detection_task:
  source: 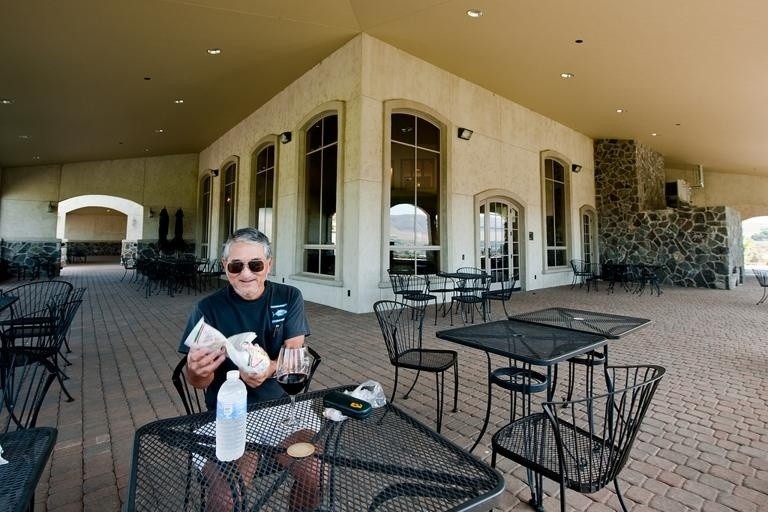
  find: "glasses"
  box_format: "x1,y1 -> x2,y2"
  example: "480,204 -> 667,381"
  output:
227,259 -> 265,274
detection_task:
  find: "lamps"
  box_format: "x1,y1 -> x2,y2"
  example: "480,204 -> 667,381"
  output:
571,163 -> 581,173
210,168 -> 218,177
457,126 -> 472,140
147,206 -> 153,218
278,131 -> 291,144
46,200 -> 53,212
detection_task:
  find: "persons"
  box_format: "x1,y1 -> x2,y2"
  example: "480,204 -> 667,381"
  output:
178,227 -> 329,512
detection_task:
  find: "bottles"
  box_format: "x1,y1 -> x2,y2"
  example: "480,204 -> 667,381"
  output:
215,369 -> 248,462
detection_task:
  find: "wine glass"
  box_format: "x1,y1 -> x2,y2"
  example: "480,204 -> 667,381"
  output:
276,343 -> 311,426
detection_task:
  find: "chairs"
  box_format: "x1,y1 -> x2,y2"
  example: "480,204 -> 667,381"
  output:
120,249 -> 224,300
2,281 -> 87,430
172,343 -> 322,512
491,365 -> 665,510
570,259 -> 664,298
387,267 -> 492,328
482,273 -> 519,320
751,266 -> 767,308
372,300 -> 458,434
